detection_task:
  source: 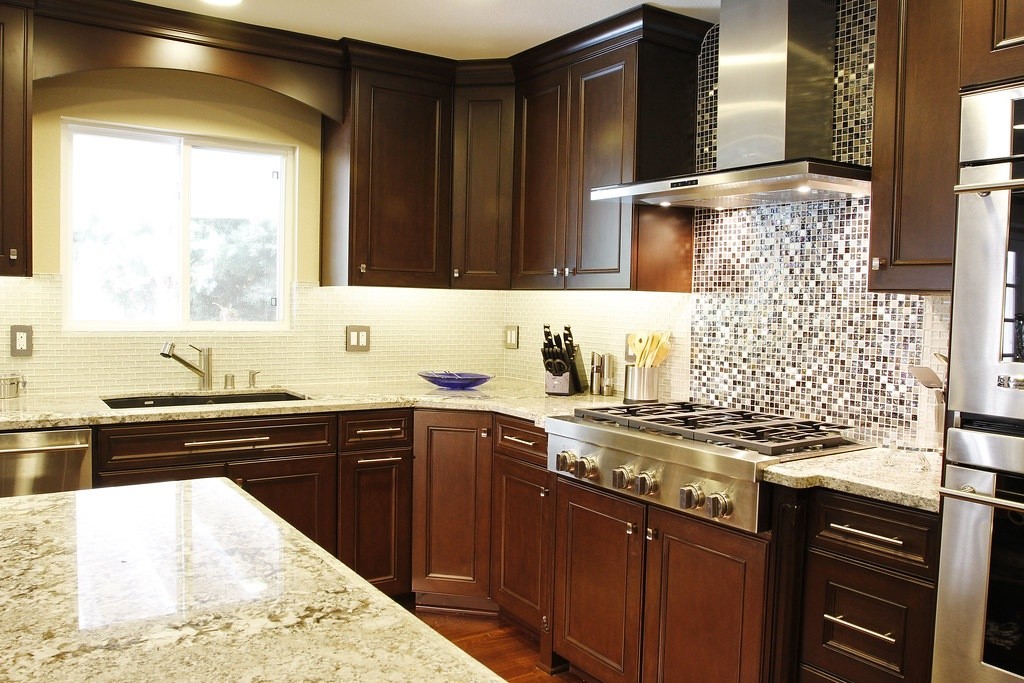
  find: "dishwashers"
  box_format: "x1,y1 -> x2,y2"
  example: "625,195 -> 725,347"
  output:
0,426 -> 93,497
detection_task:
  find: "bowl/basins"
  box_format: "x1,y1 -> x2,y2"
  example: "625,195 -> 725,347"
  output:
417,372 -> 492,389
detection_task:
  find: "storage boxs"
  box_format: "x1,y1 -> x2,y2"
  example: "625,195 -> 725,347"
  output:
543,344 -> 579,395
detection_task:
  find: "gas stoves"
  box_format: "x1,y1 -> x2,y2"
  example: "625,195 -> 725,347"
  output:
544,400 -> 856,534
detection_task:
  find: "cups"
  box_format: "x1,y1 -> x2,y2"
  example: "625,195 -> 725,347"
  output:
623,365 -> 659,404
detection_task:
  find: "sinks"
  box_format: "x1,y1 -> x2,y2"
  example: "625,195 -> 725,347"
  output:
100,386 -> 312,409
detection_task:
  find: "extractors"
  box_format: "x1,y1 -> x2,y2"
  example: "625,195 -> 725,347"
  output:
590,156 -> 871,210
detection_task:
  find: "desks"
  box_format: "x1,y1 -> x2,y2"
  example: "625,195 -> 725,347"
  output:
0,476 -> 510,683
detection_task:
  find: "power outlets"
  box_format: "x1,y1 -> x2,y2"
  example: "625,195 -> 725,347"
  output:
624,333 -> 637,363
504,325 -> 519,349
346,325 -> 370,352
10,325 -> 32,357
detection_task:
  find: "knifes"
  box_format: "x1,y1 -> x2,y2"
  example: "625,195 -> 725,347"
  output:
540,323 -> 575,371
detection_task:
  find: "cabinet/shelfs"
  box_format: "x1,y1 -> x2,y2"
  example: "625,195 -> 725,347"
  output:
491,412 -> 570,674
552,475 -> 775,683
336,409 -> 413,595
866,0 -> 962,296
449,59 -> 514,292
316,37 -> 452,291
957,0 -> 1024,92
794,487 -> 939,683
0,0 -> 33,279
510,2 -> 718,294
92,410 -> 338,558
410,407 -> 499,618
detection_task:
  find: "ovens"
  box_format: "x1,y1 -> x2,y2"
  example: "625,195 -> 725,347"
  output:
930,78 -> 1024,683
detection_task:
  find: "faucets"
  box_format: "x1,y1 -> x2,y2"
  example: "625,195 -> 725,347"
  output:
249,370 -> 260,387
160,340 -> 213,390
